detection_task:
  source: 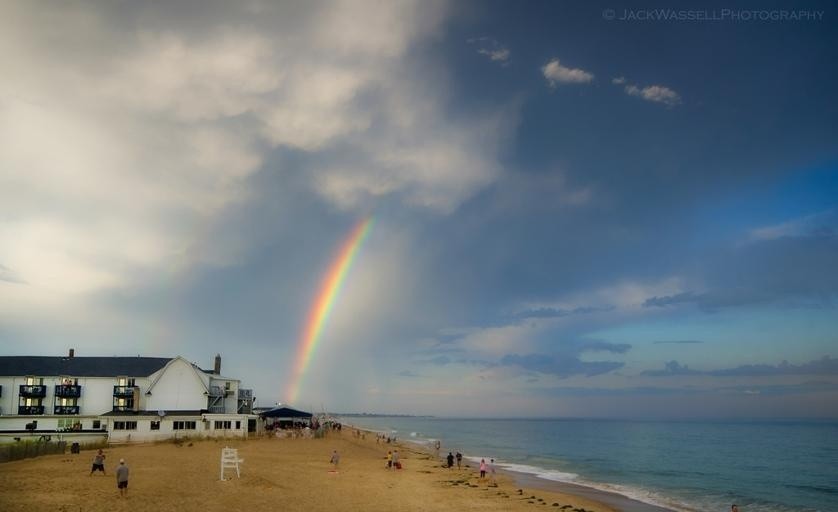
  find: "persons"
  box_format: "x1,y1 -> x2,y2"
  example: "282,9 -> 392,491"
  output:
329,449 -> 340,472
479,458 -> 497,485
88,449 -> 107,480
385,450 -> 402,473
447,451 -> 462,472
263,415 -> 341,440
351,424 -> 397,444
116,458 -> 129,499
